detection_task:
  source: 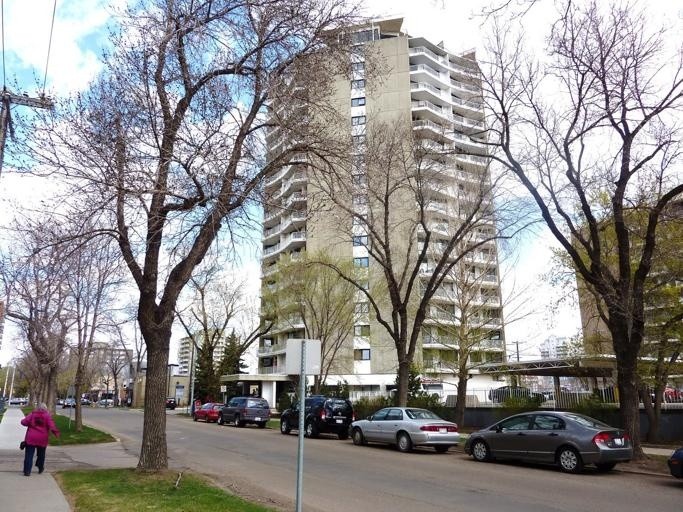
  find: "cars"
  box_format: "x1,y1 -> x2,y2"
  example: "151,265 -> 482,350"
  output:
347,407 -> 463,453
9,394 -> 116,409
668,448 -> 683,479
550,387 -> 570,399
489,385 -> 546,407
193,402 -> 224,423
651,387 -> 683,403
463,410 -> 634,474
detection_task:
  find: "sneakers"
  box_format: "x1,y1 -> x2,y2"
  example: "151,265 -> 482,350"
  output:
40,468 -> 43,473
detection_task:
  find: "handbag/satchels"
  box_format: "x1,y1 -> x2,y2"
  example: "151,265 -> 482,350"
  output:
21,442 -> 25,449
24,473 -> 29,476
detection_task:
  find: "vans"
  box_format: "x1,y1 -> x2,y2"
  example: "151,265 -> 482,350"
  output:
218,396 -> 271,428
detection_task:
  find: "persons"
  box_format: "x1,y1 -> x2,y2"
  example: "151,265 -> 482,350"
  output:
253,388 -> 259,397
19,402 -> 60,476
190,400 -> 196,419
252,389 -> 255,395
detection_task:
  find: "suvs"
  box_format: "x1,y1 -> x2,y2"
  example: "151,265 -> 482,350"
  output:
280,394 -> 354,440
165,397 -> 177,410
